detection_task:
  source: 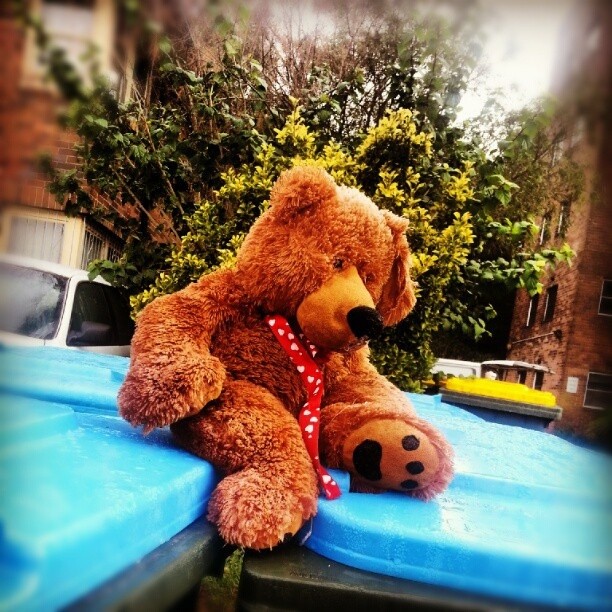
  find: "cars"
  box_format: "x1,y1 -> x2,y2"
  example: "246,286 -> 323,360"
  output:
0,251 -> 134,358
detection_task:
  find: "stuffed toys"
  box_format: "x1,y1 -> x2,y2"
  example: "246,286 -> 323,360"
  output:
116,163 -> 455,551
